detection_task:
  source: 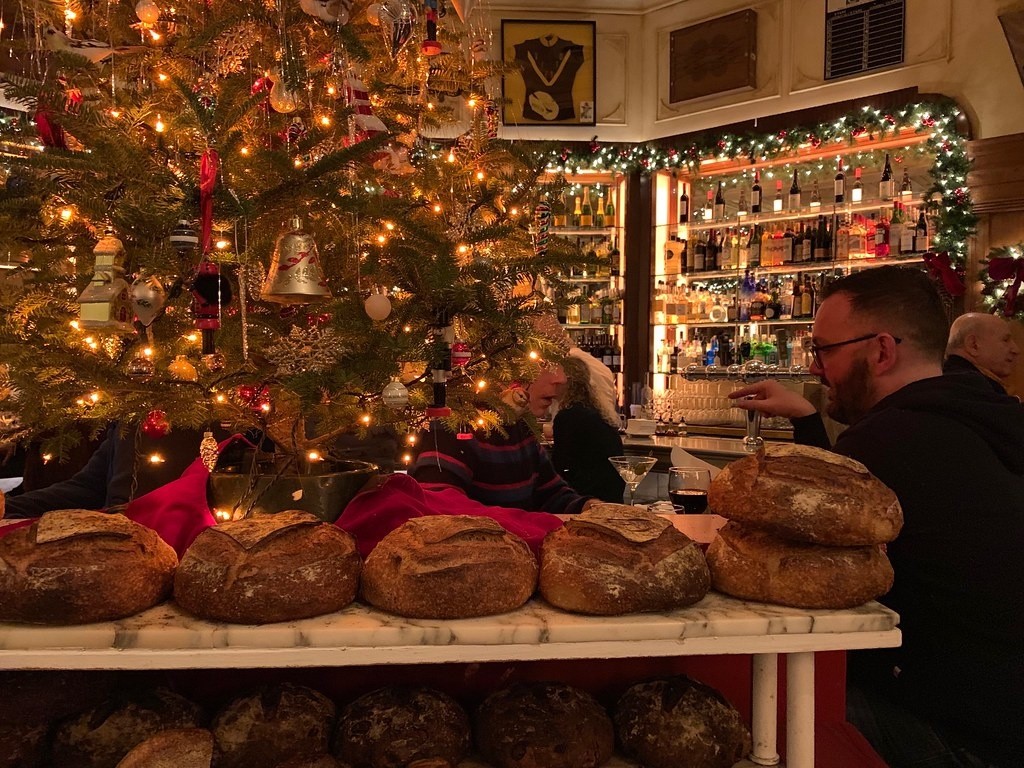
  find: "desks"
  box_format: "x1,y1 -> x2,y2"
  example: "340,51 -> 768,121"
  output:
1,597 -> 905,767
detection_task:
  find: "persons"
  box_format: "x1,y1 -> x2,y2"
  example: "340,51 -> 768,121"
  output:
409,307 -> 625,512
0,416 -> 277,520
728,267 -> 1024,768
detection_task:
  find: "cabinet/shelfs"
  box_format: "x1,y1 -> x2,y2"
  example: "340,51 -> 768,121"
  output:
647,129 -> 961,441
529,170 -> 627,427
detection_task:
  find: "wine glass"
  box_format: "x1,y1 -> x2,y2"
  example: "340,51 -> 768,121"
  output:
646,375 -> 747,428
608,456 -> 658,507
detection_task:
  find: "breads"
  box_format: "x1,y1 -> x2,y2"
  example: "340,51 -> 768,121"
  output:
0,672 -> 755,768
703,442 -> 902,610
0,504 -> 713,626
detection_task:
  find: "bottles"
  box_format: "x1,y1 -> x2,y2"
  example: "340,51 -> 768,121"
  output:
837,202 -> 892,261
704,167 -> 918,220
739,190 -> 748,211
713,181 -> 725,224
657,326 -> 814,375
751,171 -> 762,213
574,333 -> 622,373
788,169 -> 802,213
545,284 -> 621,327
834,159 -> 846,204
613,373 -> 687,437
538,186 -> 615,229
680,183 -> 689,223
651,265 -> 854,324
534,235 -> 620,280
665,216 -> 832,274
879,154 -> 894,201
889,201 -> 944,258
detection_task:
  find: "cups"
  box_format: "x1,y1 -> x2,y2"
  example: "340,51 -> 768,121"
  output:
669,467 -> 711,515
647,504 -> 685,515
630,405 -> 640,419
543,425 -> 554,441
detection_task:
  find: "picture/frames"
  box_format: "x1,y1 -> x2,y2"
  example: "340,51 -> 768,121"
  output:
669,9 -> 759,105
501,17 -> 596,127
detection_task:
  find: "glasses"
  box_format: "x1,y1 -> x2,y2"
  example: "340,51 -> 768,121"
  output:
808,334 -> 903,370
